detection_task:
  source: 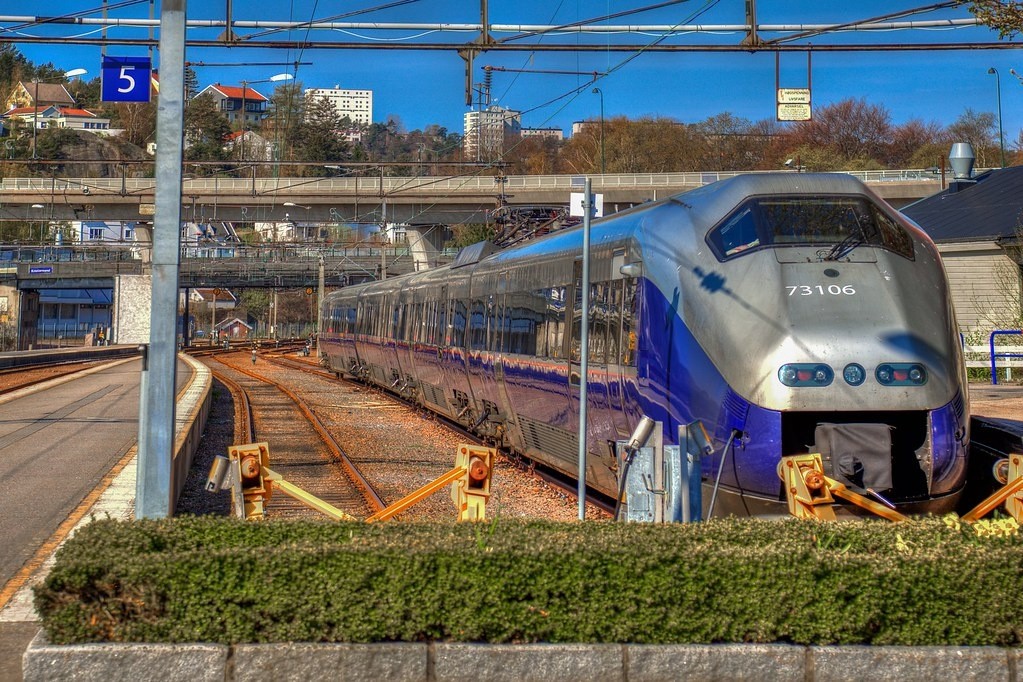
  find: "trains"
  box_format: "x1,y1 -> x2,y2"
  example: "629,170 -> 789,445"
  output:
314,172 -> 972,520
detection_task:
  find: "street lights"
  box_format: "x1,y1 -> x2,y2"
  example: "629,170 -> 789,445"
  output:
592,87 -> 606,174
241,73 -> 295,162
28,68 -> 89,162
987,67 -> 1005,167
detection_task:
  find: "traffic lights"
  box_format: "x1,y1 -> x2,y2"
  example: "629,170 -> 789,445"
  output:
212,287 -> 221,297
306,288 -> 313,295
